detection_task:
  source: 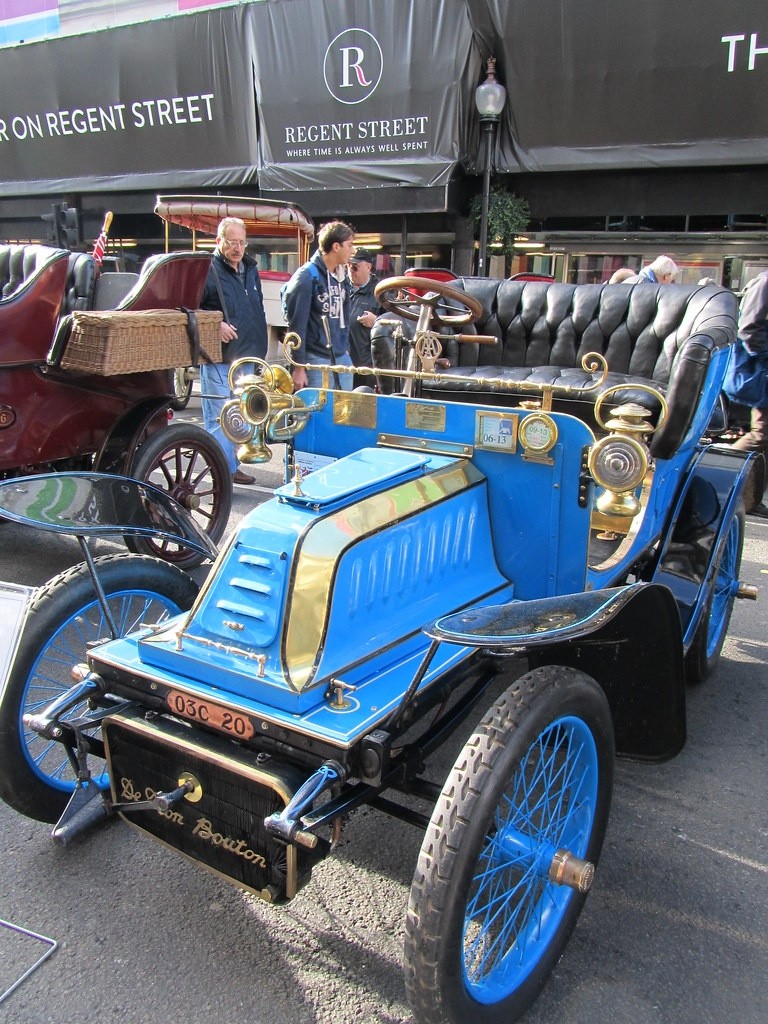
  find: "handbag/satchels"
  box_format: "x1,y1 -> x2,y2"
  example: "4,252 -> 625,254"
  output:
722,340 -> 768,408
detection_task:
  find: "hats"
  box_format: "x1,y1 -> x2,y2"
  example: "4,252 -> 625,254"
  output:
347,247 -> 372,264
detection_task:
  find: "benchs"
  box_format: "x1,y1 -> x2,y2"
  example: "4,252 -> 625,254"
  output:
0,244 -> 71,368
114,250 -> 213,310
371,275 -> 739,459
59,251 -> 98,319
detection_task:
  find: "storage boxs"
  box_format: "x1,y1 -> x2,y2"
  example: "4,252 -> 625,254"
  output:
62,309 -> 223,375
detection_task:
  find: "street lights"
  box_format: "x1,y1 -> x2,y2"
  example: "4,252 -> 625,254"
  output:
474,55 -> 507,279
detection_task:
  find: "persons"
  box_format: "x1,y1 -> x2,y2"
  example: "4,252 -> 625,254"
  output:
345,249 -> 396,390
698,270 -> 768,518
602,254 -> 679,283
283,218 -> 356,390
199,215 -> 272,487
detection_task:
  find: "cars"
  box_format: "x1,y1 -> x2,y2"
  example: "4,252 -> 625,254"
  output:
0,276 -> 768,1024
0,244 -> 227,572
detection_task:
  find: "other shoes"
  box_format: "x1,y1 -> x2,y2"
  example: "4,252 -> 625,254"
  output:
233,470 -> 256,484
745,503 -> 768,518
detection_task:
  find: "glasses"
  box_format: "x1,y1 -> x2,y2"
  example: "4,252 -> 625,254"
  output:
346,263 -> 368,271
225,239 -> 248,247
